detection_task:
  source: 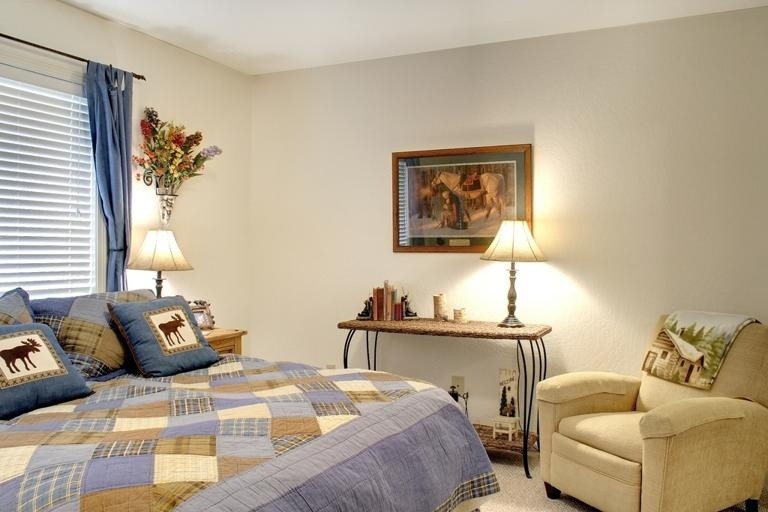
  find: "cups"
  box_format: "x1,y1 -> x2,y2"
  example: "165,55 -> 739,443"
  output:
433,294 -> 445,320
452,307 -> 466,321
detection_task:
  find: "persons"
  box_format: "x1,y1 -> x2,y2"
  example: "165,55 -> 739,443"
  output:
441,190 -> 462,230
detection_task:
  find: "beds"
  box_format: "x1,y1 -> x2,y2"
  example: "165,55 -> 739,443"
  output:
1,277 -> 504,512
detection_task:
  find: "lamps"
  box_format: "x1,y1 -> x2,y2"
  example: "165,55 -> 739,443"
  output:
478,217 -> 546,330
128,228 -> 195,298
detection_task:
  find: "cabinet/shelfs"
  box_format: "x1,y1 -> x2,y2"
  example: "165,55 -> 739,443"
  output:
201,326 -> 250,355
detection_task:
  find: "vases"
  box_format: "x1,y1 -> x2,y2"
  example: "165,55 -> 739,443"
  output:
159,193 -> 175,226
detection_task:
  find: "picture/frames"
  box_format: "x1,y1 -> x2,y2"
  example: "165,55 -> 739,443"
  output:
386,141 -> 535,258
191,306 -> 216,331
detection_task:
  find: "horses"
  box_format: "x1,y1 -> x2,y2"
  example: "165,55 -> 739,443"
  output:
431,170 -> 506,221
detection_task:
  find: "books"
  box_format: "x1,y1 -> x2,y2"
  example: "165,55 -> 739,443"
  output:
372,279 -> 407,321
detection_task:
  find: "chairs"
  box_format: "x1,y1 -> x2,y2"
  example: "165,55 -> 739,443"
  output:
532,304 -> 767,511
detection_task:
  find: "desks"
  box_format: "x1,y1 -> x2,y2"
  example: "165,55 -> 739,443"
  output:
336,314 -> 550,480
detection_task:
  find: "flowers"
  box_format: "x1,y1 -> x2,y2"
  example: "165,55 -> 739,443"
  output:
128,102 -> 224,195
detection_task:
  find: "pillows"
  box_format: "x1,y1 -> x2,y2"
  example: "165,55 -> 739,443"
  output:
31,288 -> 155,380
101,293 -> 223,376
2,320 -> 94,418
0,286 -> 34,327
